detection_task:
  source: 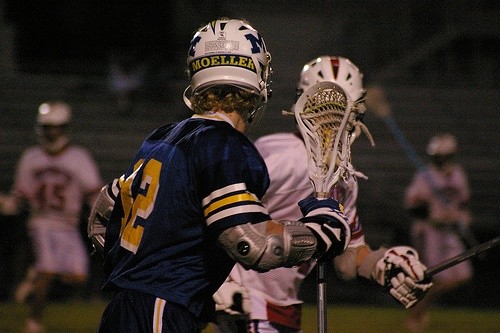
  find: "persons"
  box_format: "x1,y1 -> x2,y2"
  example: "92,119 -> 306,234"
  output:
404,133 -> 473,331
87,16 -> 350,332
212,54 -> 432,332
1,98 -> 103,332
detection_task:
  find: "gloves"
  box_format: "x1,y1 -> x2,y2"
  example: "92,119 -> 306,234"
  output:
373,245 -> 433,310
298,197 -> 351,262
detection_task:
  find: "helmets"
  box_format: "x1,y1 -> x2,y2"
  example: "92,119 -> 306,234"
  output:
34,102 -> 71,153
185,18 -> 271,110
428,135 -> 457,172
296,55 -> 367,144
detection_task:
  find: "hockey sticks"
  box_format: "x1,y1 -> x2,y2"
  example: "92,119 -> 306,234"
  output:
423,237 -> 500,278
293,80 -> 356,333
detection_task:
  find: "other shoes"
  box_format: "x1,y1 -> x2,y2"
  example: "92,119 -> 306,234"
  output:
27,317 -> 41,333
16,270 -> 39,306
